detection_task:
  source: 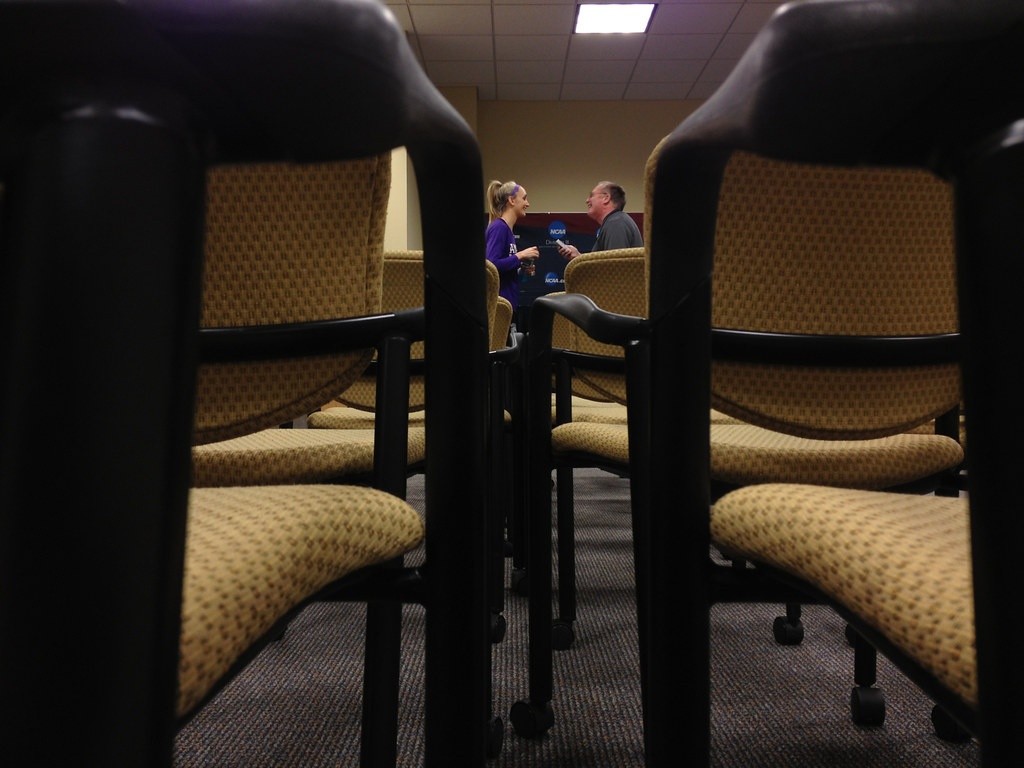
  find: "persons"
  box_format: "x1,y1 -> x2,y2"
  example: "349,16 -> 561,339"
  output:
485,179 -> 540,348
558,180 -> 644,258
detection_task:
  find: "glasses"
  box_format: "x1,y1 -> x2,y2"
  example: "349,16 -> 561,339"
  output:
588,192 -> 608,198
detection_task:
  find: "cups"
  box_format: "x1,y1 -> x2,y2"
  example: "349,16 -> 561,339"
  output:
521,258 -> 534,269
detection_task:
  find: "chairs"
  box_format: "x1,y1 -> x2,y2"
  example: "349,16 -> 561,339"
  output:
1,0 -> 1024,768
